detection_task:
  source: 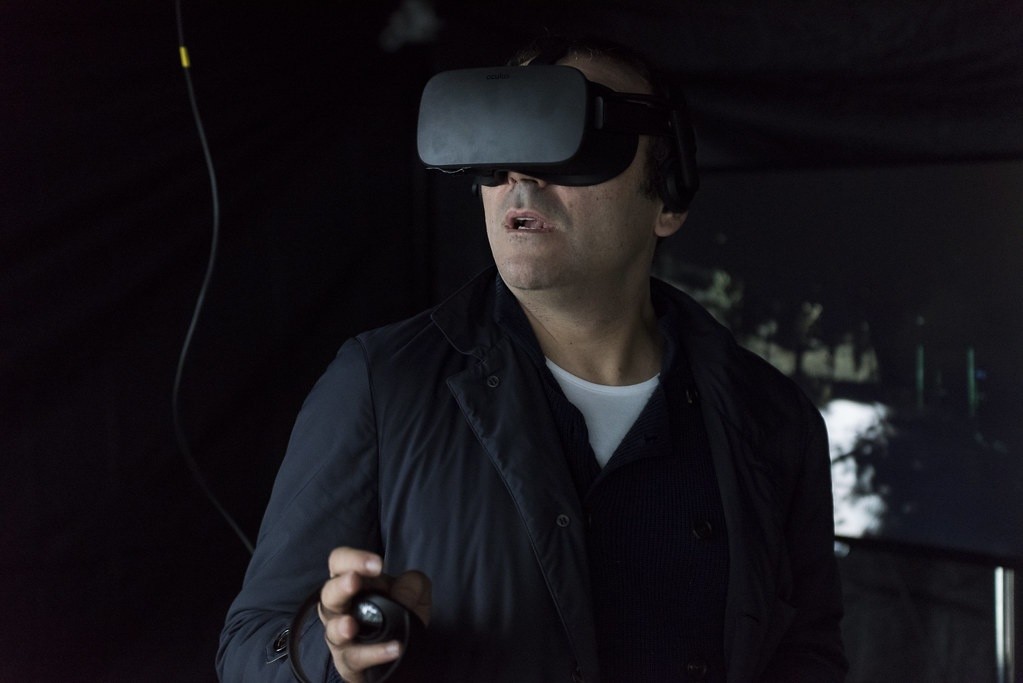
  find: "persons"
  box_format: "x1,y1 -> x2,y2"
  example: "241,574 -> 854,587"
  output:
213,24 -> 849,682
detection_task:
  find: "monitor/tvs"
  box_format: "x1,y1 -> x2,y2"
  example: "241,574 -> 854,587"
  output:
651,151 -> 1022,572
